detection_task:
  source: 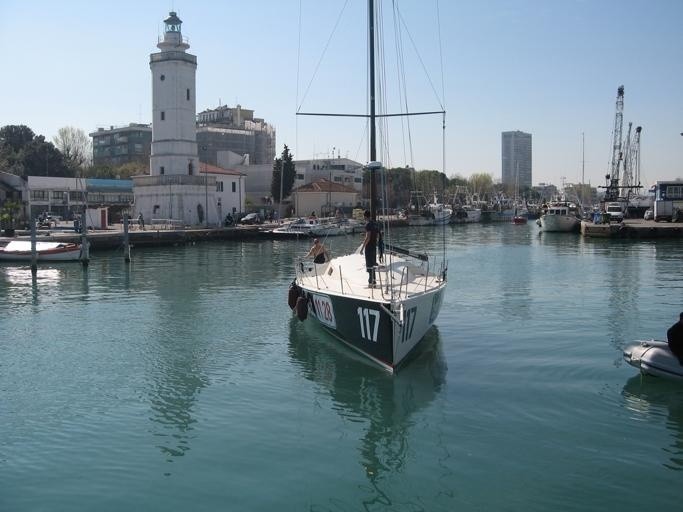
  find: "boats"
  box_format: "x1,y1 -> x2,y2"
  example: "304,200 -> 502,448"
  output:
0,240 -> 89,261
622,339 -> 682,380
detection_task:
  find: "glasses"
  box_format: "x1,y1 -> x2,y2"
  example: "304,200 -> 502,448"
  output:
314,241 -> 319,244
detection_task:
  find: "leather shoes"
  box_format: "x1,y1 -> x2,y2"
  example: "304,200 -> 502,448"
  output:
368,284 -> 376,287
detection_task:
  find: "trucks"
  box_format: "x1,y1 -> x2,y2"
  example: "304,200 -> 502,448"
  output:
652,200 -> 672,222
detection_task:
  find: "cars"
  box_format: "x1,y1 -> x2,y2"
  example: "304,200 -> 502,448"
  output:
603,205 -> 624,223
240,213 -> 264,225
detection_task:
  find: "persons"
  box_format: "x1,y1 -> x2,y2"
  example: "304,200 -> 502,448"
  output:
224,212 -> 233,226
298,237 -> 329,276
359,209 -> 382,291
273,209 -> 278,223
310,211 -> 317,224
335,207 -> 344,227
666,312 -> 683,367
136,212 -> 145,230
